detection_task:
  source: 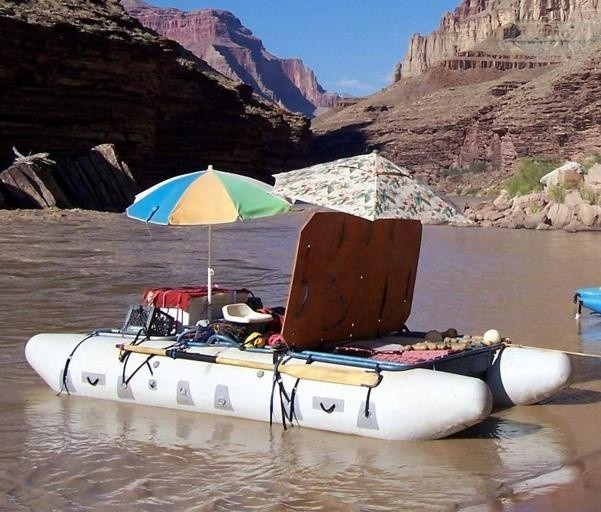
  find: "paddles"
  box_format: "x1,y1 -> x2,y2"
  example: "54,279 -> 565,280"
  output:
116,342 -> 383,388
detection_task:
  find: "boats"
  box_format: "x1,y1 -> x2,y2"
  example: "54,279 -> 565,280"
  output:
25,304 -> 574,442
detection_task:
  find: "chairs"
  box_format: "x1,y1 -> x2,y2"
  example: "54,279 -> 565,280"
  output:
222,302 -> 274,324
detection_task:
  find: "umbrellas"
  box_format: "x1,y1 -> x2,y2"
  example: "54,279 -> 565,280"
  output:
267,148 -> 480,230
125,165 -> 296,322
